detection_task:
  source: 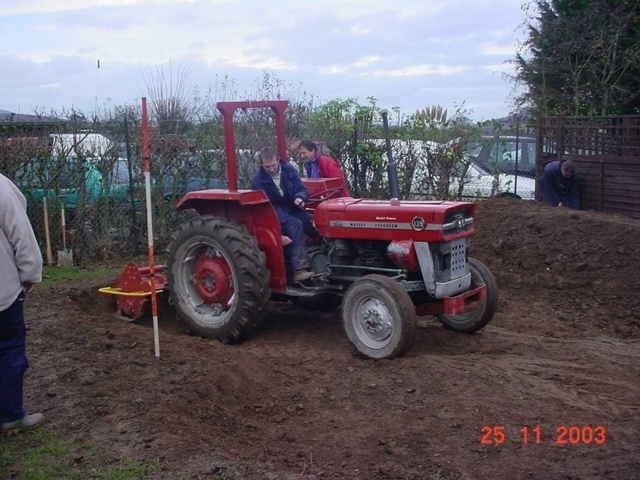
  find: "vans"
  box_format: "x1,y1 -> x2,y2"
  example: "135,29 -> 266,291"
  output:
161,149 -> 261,200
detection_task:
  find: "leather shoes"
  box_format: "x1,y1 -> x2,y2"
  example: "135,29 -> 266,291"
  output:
0,412 -> 45,433
293,270 -> 315,282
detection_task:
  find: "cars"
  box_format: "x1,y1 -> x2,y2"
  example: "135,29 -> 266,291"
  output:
14,155 -> 157,226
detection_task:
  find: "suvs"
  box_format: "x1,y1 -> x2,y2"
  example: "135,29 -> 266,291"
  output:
442,135 -> 571,176
343,138 -> 534,201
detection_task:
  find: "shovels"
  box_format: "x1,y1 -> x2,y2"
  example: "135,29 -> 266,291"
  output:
56,202 -> 74,267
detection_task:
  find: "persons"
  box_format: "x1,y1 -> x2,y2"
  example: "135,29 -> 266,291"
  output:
0,171 -> 48,437
536,157 -> 580,210
250,146 -> 322,284
297,139 -> 351,198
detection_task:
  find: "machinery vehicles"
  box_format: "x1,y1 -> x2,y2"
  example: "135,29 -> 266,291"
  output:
166,101 -> 498,359
